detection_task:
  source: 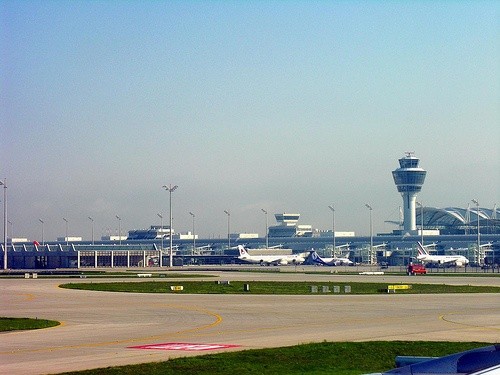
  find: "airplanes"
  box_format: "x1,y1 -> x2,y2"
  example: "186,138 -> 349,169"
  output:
416,241 -> 470,267
307,248 -> 354,266
237,245 -> 306,266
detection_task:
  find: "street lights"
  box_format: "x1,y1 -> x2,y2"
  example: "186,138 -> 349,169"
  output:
365,203 -> 374,265
328,205 -> 335,258
416,201 -> 424,247
157,213 -> 164,268
224,209 -> 231,248
261,208 -> 268,248
0,176 -> 8,269
162,184 -> 179,266
63,217 -> 69,246
472,199 -> 481,265
189,211 -> 196,255
116,215 -> 122,246
88,215 -> 95,245
38,218 -> 44,245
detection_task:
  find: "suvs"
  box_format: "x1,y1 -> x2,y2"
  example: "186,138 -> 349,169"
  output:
406,264 -> 427,275
